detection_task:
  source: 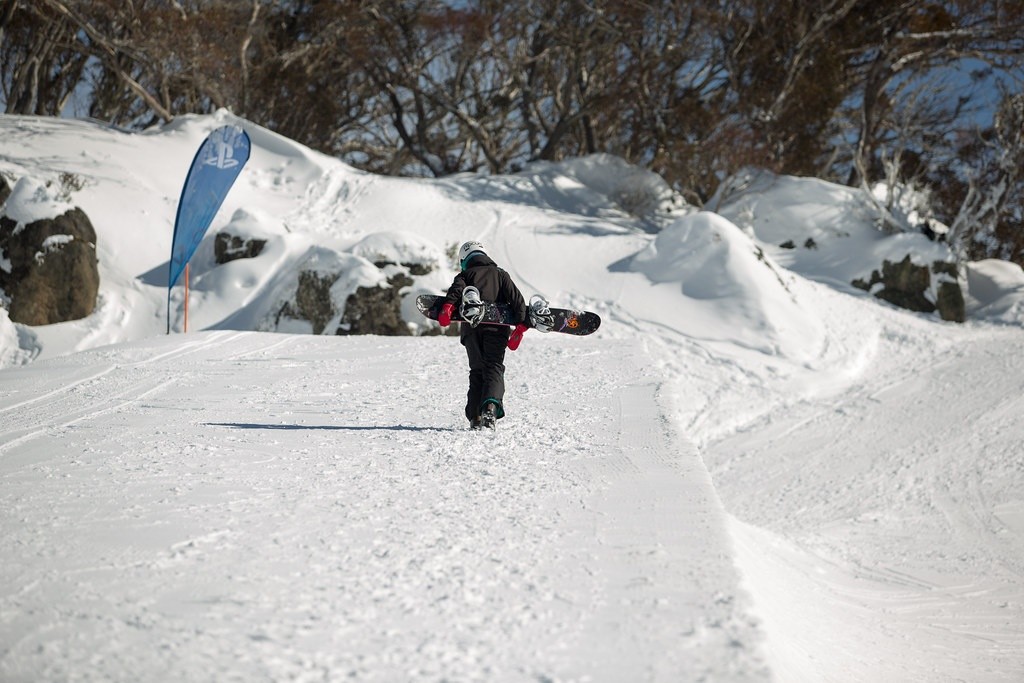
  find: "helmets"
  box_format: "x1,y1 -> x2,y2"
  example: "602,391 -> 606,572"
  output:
456,241 -> 486,266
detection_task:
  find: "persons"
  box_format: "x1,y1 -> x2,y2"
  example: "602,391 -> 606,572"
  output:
438,241 -> 530,429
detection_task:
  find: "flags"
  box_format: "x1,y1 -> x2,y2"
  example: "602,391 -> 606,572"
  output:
168,124 -> 252,288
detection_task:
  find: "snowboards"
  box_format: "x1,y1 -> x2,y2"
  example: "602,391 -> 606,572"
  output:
415,286 -> 601,337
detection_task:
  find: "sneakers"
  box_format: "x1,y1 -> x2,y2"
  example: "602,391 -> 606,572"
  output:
480,404 -> 495,431
470,414 -> 480,430
